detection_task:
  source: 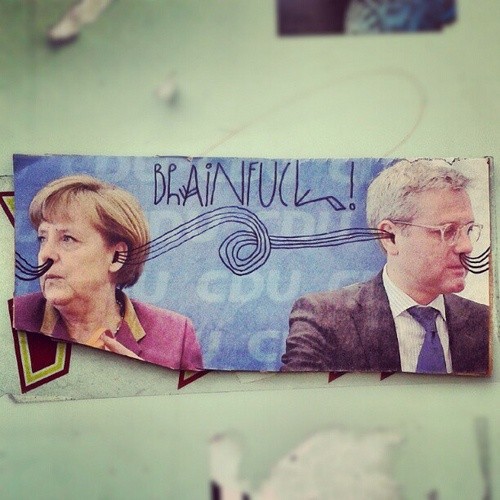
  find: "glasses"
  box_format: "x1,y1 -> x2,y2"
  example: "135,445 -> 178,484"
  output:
388,215 -> 485,248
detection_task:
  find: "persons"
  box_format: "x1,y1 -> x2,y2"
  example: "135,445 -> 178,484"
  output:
13,174 -> 203,371
278,159 -> 489,375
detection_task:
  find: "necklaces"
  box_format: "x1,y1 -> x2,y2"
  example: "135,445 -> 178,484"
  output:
113,299 -> 125,338
